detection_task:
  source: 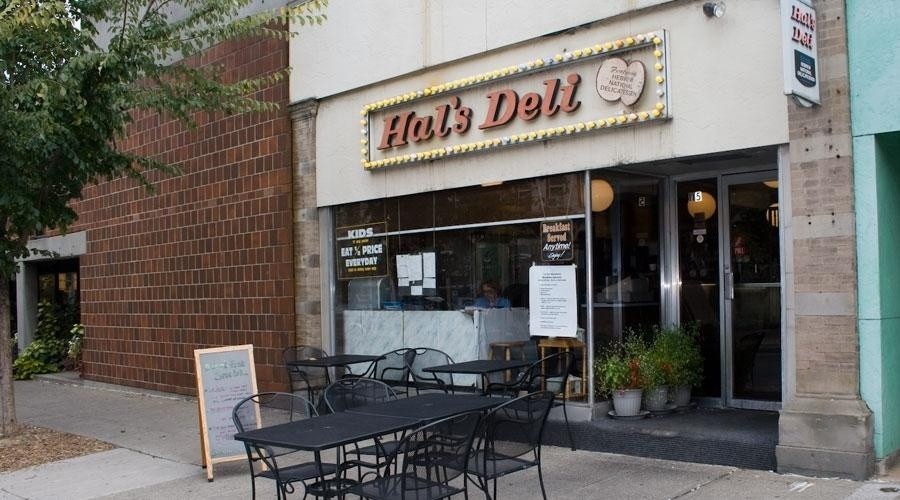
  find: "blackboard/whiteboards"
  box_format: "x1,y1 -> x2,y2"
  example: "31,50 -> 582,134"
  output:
194,343 -> 266,463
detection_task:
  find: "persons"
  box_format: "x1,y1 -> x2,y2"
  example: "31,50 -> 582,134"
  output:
472,280 -> 511,309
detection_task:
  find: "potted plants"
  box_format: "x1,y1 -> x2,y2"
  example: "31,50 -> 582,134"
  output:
593,318 -> 707,422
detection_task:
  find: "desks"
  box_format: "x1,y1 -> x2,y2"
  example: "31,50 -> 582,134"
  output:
537,340 -> 586,402
